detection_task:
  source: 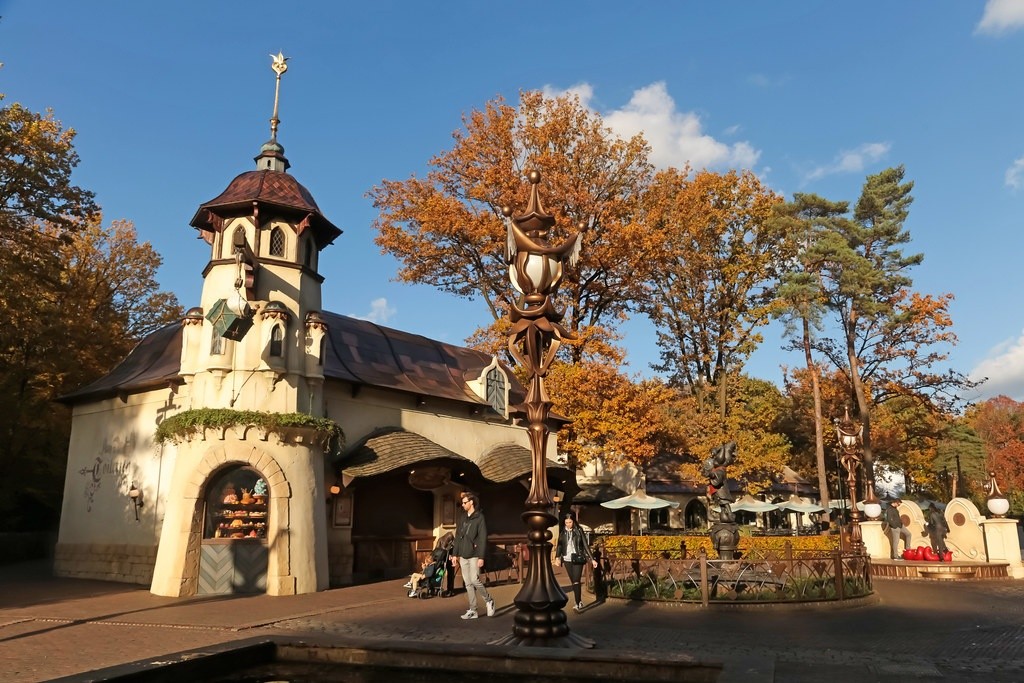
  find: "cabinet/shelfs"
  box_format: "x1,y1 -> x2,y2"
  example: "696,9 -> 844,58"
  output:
217,504 -> 267,529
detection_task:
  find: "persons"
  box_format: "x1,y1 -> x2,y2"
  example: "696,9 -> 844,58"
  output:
859,512 -> 867,521
435,533 -> 454,596
697,441 -> 737,522
555,513 -> 597,613
776,520 -> 784,529
887,499 -> 911,561
452,495 -> 496,619
925,504 -> 950,560
1009,512 -> 1024,532
403,557 -> 434,597
835,513 -> 843,527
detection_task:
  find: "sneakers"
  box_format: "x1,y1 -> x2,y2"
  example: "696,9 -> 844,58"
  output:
486,599 -> 495,616
460,608 -> 478,619
409,590 -> 417,597
403,582 -> 412,588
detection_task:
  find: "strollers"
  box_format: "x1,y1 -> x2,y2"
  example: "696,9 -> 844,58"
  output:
407,548 -> 452,600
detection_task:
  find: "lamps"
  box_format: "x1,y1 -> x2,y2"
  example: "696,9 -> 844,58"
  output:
864,479 -> 882,521
130,481 -> 144,521
329,470 -> 341,495
987,470 -> 1010,518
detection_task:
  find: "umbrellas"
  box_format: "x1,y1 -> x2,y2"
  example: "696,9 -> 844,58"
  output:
819,500 -> 947,510
776,497 -> 826,534
712,494 -> 780,525
601,490 -> 680,536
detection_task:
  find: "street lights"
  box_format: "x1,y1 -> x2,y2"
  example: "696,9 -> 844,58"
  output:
485,168 -> 602,650
834,406 -> 869,555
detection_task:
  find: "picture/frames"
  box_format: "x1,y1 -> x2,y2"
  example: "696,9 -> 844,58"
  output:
332,493 -> 353,528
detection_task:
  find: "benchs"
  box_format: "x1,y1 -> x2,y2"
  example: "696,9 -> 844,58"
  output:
480,540 -> 516,585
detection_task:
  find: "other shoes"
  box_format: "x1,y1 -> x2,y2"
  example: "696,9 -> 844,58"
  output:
445,590 -> 455,596
573,605 -> 580,614
579,601 -> 584,609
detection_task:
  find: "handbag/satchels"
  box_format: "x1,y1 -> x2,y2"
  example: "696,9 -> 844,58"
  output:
571,553 -> 587,565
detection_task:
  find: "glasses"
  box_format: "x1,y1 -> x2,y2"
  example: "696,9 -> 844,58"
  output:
462,501 -> 470,505
